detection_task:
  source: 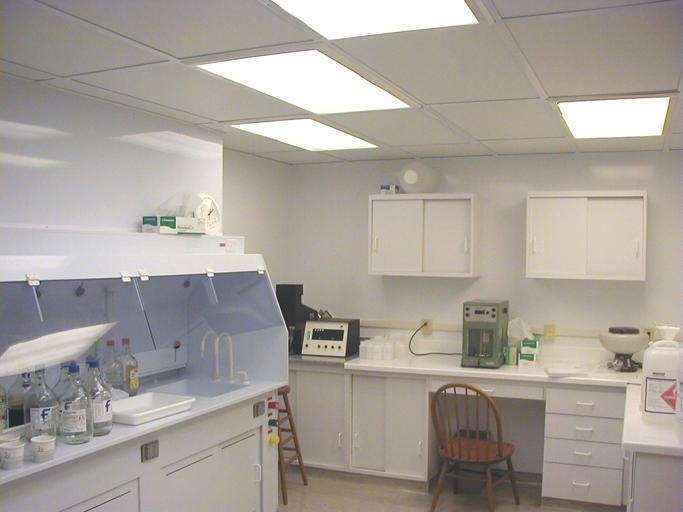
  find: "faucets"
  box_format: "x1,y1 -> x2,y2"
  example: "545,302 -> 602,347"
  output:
200,330 -> 220,376
214,332 -> 234,379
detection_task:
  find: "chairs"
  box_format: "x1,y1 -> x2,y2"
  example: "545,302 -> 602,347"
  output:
430,382 -> 520,512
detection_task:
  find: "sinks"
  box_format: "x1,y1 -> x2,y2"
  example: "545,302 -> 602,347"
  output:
144,378 -> 247,399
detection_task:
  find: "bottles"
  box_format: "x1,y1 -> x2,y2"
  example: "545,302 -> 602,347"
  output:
0,336 -> 140,445
357,334 -> 406,362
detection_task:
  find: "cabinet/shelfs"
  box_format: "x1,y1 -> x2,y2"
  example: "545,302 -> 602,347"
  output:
542,385 -> 626,508
368,195 -> 475,279
1,405 -> 268,511
527,192 -> 646,280
281,369 -> 443,481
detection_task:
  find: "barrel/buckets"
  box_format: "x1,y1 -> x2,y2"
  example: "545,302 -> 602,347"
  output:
640,340 -> 682,421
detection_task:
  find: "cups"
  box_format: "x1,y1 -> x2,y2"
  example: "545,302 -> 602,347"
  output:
0,432 -> 24,470
29,435 -> 56,464
